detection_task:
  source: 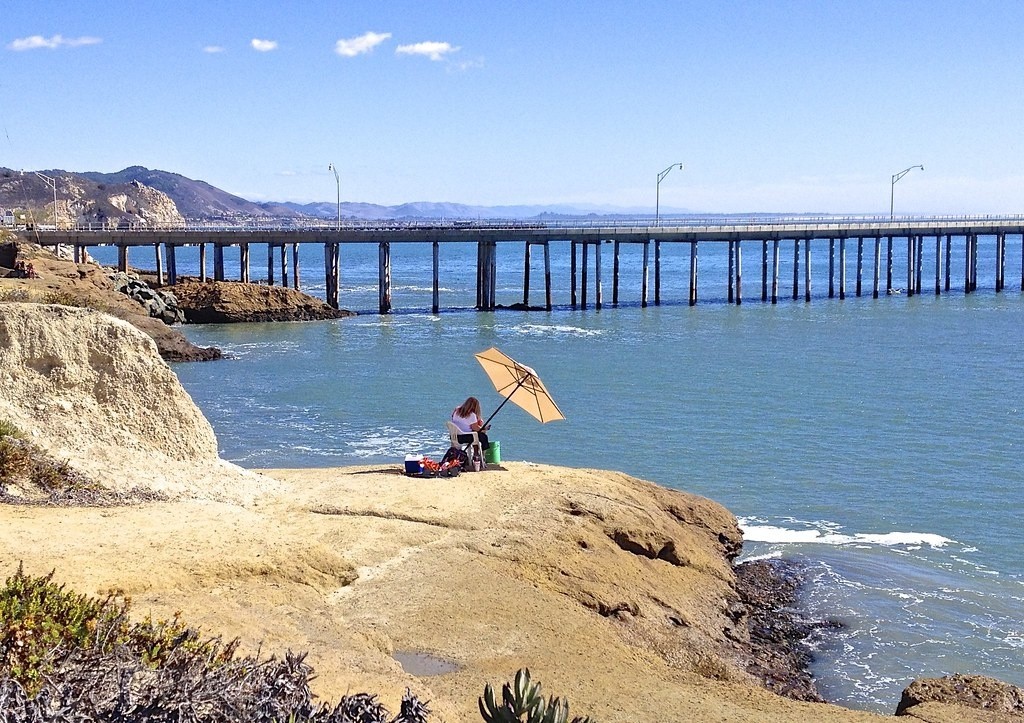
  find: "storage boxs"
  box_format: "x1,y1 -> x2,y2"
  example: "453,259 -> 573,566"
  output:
405,453 -> 423,474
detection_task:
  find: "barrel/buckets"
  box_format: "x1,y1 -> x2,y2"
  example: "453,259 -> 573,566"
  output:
485,441 -> 501,464
405,454 -> 424,475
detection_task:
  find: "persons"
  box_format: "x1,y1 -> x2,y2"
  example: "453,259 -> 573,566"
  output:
450,397 -> 489,468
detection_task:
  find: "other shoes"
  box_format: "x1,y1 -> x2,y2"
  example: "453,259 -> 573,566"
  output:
482,462 -> 489,468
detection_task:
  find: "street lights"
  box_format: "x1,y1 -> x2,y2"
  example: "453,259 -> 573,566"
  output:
890,165 -> 924,223
655,162 -> 683,227
328,163 -> 341,231
19,167 -> 58,231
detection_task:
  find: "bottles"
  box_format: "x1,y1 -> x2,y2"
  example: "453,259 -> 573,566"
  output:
474,457 -> 481,472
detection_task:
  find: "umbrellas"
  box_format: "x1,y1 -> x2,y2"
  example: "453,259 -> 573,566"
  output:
464,347 -> 566,452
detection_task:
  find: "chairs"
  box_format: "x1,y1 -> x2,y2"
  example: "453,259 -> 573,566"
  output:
446,421 -> 485,469
